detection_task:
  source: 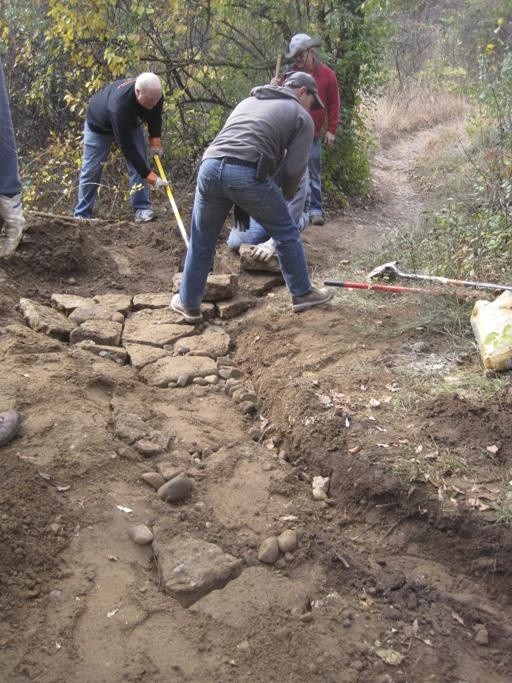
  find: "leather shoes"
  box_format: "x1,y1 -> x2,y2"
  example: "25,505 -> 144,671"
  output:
291,286 -> 334,311
170,292 -> 204,323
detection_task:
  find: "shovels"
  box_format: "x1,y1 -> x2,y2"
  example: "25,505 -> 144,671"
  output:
369,260 -> 512,291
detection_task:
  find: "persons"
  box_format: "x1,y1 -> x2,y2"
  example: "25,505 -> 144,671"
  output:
270,33 -> 340,225
169,71 -> 334,324
227,148 -> 311,262
74,71 -> 172,224
0,70 -> 27,262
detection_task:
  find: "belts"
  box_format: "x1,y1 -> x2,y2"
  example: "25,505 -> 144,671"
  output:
219,156 -> 258,169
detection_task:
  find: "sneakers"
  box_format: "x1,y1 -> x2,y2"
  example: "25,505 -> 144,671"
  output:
311,215 -> 325,225
134,208 -> 155,222
0,410 -> 20,447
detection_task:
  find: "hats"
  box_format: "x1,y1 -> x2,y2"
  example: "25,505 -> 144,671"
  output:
284,72 -> 325,111
285,33 -> 321,59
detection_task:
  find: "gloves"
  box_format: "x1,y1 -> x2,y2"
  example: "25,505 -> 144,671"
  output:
234,204 -> 249,232
0,194 -> 25,257
149,137 -> 163,162
145,172 -> 174,197
250,237 -> 277,263
